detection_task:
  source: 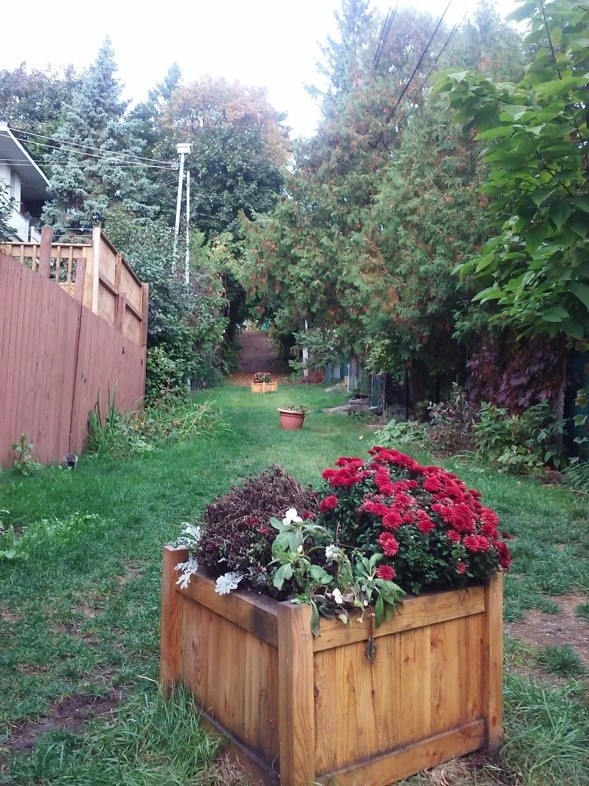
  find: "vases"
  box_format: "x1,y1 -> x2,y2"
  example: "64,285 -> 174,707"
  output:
349,398 -> 367,405
160,544 -> 504,786
251,381 -> 278,394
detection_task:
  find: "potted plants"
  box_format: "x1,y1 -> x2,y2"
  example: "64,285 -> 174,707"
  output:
276,404 -> 308,430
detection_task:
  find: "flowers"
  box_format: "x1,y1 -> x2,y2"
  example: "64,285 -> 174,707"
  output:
162,443 -> 516,637
253,371 -> 272,384
348,393 -> 369,401
